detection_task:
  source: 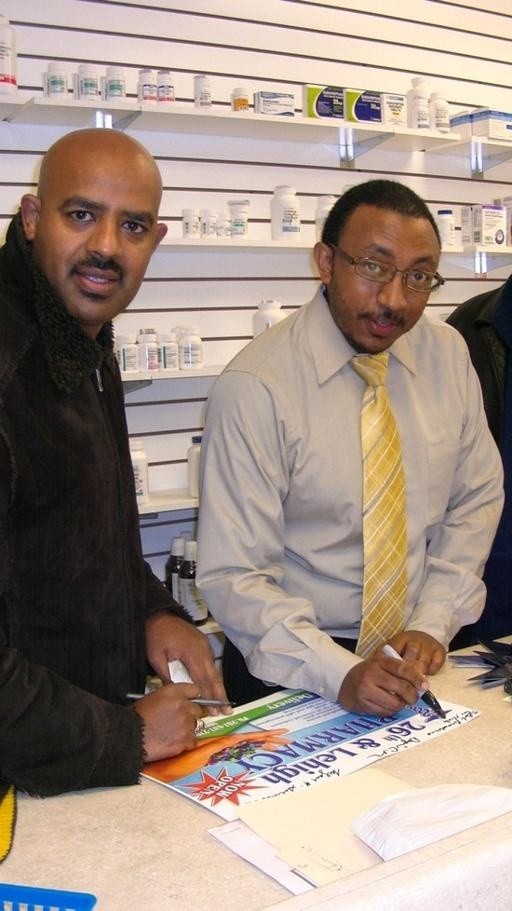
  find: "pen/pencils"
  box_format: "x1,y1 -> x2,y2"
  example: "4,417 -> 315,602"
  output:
382,644 -> 446,719
125,693 -> 236,705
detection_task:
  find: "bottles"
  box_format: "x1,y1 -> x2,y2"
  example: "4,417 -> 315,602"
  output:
185,436 -> 204,503
183,185 -> 338,239
115,327 -> 204,373
407,76 -> 450,131
0,12 -> 250,113
435,208 -> 460,248
167,538 -> 208,625
129,440 -> 147,506
253,299 -> 282,334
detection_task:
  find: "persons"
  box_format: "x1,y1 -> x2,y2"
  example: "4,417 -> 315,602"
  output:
195,181 -> 505,717
0,128 -> 234,797
444,273 -> 512,652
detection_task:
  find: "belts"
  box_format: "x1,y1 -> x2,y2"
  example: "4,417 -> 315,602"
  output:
330,636 -> 357,652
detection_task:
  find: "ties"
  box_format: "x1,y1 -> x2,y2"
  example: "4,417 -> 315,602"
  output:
351,351 -> 408,660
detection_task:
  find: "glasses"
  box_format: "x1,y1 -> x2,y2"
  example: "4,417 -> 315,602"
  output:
330,241 -> 446,293
